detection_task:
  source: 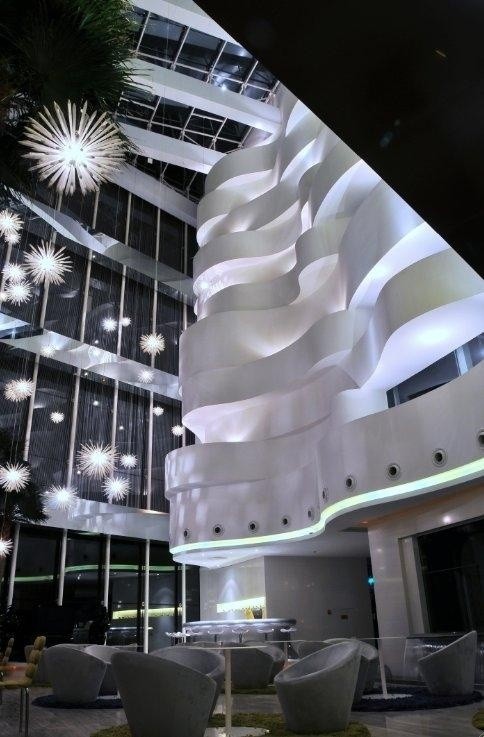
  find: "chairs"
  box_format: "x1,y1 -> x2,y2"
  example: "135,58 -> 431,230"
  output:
296,638 -> 335,657
275,637 -> 363,733
415,626 -> 481,698
325,636 -> 380,695
25,635 -> 287,737
